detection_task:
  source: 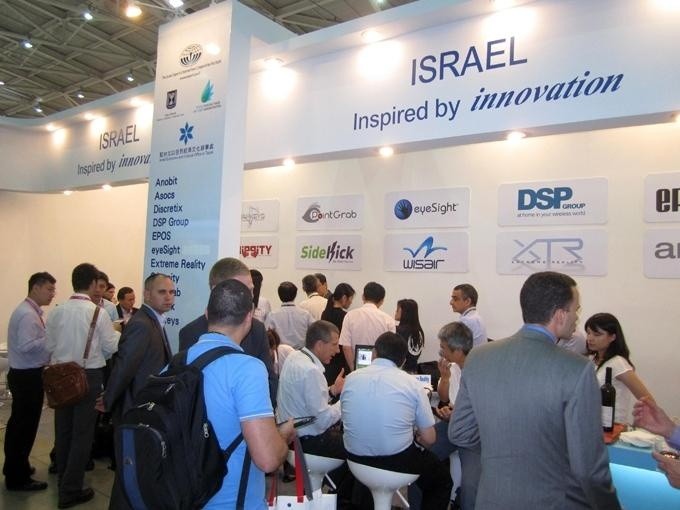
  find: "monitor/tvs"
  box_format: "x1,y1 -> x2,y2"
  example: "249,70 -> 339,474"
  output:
351,343 -> 375,371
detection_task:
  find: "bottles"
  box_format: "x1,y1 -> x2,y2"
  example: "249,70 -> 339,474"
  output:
598,368 -> 615,432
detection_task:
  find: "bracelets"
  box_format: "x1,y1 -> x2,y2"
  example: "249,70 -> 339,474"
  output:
328,386 -> 336,399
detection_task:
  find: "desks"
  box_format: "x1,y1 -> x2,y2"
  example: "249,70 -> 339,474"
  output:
602,423 -> 680,510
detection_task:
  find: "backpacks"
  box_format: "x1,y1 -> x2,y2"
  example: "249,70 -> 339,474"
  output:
109,367 -> 230,510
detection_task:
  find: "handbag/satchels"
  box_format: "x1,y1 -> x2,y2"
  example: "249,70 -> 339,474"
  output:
265,487 -> 338,510
43,360 -> 90,409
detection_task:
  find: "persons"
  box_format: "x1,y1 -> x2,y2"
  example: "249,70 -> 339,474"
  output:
448,271 -> 623,509
276,320 -> 347,460
91,271 -> 119,320
44,263 -> 122,507
408,322 -> 472,509
178,257 -> 277,410
102,283 -> 116,300
312,272 -> 332,298
158,279 -> 298,510
555,329 -> 587,354
265,328 -> 295,376
94,273 -> 175,510
450,284 -> 488,347
116,287 -> 138,320
585,313 -> 658,426
395,299 -> 424,374
264,281 -> 318,348
339,282 -> 396,371
3,271 -> 57,490
632,397 -> 680,489
340,332 -> 453,510
299,274 -> 328,319
321,283 -> 356,386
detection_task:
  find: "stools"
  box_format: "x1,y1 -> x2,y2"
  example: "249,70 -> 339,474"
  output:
347,459 -> 420,510
0,351 -> 13,399
0,356 -> 9,406
284,449 -> 345,496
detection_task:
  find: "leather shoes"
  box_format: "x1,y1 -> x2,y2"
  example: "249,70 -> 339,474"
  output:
2,466 -> 37,477
4,478 -> 49,492
56,488 -> 94,510
